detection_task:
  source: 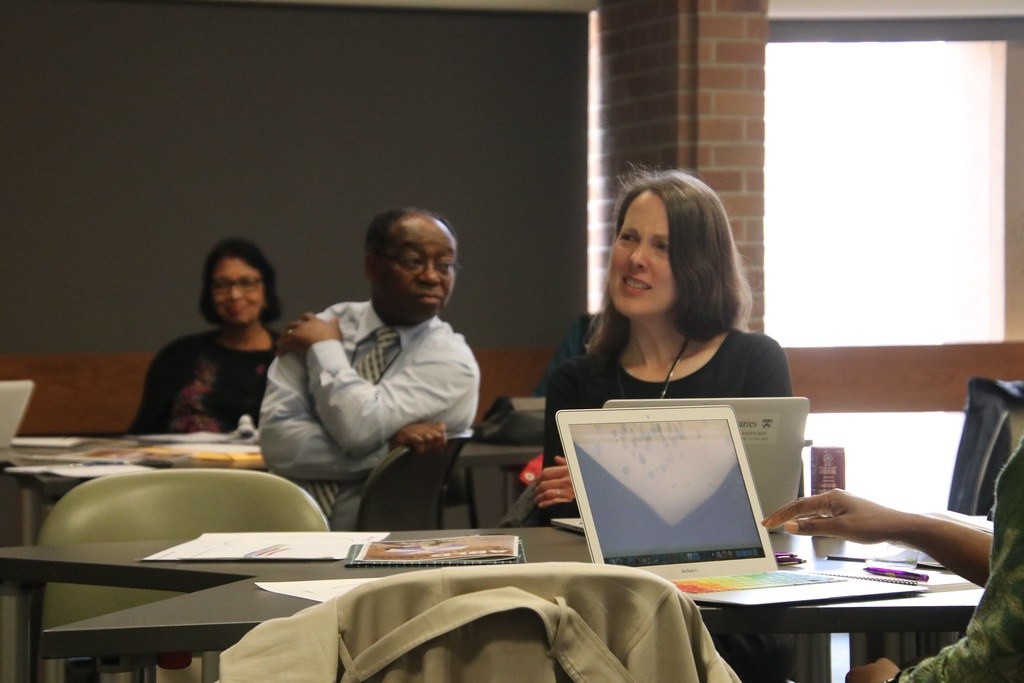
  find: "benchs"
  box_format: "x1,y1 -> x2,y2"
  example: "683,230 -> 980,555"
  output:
0,349 -> 562,435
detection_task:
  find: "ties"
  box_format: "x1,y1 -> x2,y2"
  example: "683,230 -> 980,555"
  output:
301,325 -> 399,518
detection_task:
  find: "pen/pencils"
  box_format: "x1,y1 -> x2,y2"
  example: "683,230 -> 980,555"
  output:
863,567 -> 929,583
776,552 -> 797,558
777,558 -> 807,565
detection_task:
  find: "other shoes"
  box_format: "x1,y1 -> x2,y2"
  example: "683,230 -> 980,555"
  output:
157,651 -> 193,670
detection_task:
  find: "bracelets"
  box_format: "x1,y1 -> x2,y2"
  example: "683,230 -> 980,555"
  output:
888,671 -> 902,683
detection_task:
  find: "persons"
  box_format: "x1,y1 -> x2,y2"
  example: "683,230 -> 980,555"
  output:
258,207 -> 481,531
126,237 -> 282,436
761,438 -> 1024,683
500,170 -> 793,529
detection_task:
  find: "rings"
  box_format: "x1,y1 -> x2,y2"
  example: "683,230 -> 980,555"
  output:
287,329 -> 292,335
557,489 -> 560,497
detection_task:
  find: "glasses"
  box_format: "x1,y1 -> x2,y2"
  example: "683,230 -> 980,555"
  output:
371,249 -> 463,280
213,277 -> 261,295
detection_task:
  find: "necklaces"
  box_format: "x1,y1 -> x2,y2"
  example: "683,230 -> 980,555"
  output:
657,337 -> 689,399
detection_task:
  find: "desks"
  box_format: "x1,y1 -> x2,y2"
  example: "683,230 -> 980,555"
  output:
0,432 -> 544,546
0,524 -> 990,683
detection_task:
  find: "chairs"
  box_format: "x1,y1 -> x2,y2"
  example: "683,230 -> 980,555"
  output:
356,427 -> 475,531
31,467 -> 328,683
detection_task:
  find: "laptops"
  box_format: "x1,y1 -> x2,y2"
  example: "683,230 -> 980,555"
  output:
551,397 -> 929,608
0,379 -> 35,454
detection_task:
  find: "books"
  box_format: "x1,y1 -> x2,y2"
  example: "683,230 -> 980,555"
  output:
344,535 -> 527,569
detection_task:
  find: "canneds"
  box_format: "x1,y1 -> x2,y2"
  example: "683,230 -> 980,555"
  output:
810,446 -> 846,495
